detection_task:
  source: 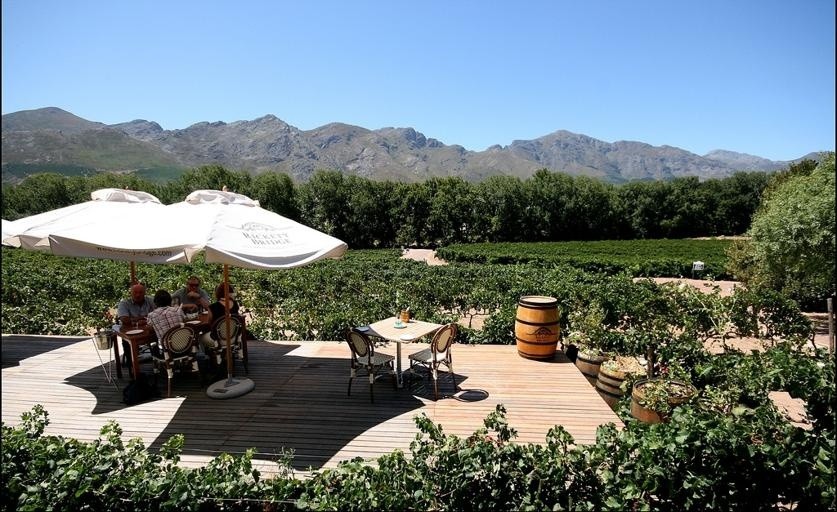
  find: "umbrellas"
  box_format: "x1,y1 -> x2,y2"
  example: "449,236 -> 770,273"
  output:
0,185 -> 167,286
49,186 -> 349,378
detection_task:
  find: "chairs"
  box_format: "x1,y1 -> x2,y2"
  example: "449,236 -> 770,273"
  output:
345,328 -> 397,405
152,325 -> 202,398
122,340 -> 157,368
408,323 -> 460,401
205,314 -> 249,379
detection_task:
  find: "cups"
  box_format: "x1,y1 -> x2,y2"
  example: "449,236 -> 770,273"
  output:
400,309 -> 409,323
132,322 -> 139,330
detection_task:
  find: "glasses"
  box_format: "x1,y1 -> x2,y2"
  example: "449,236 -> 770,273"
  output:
186,281 -> 200,287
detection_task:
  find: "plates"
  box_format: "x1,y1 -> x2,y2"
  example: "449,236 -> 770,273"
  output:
355,327 -> 369,332
394,322 -> 407,329
126,330 -> 144,336
186,320 -> 202,325
400,334 -> 415,341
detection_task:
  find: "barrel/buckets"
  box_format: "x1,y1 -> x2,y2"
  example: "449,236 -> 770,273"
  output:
94,332 -> 113,350
575,353 -> 694,425
515,295 -> 560,361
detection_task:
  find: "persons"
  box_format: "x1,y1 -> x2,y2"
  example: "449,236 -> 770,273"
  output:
116,282 -> 162,371
200,282 -> 239,371
145,290 -> 188,376
169,274 -> 211,310
